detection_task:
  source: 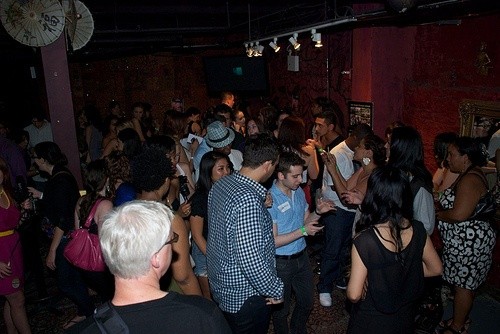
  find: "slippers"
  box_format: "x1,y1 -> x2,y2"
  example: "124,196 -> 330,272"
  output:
63,317 -> 87,330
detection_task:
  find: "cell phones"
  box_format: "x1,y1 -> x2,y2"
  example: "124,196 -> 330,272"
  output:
79,190 -> 87,197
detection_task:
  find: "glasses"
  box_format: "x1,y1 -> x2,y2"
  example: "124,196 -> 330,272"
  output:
165,232 -> 179,244
31,155 -> 41,160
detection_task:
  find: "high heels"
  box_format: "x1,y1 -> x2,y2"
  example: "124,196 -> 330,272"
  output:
435,326 -> 465,334
440,317 -> 470,331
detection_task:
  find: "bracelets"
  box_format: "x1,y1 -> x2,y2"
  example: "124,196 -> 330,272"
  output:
315,209 -> 322,216
190,157 -> 194,159
301,227 -> 307,236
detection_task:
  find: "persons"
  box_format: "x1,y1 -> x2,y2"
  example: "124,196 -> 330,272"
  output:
0,93 -> 500,334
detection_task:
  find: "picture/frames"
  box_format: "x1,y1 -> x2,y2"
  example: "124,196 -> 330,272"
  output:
348,100 -> 374,139
459,99 -> 500,174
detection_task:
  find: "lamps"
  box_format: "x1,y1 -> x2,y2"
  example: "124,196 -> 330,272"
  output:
245,42 -> 264,58
289,32 -> 301,50
269,37 -> 281,53
310,28 -> 324,47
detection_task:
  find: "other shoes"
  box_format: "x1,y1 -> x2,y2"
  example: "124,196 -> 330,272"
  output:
335,280 -> 348,290
320,293 -> 332,307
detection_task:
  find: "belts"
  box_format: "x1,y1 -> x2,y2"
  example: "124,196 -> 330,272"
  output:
275,245 -> 307,260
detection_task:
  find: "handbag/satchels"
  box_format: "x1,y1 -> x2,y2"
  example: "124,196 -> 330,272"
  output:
63,198 -> 105,272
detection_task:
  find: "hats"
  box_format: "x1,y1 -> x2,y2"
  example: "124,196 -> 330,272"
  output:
204,121 -> 235,148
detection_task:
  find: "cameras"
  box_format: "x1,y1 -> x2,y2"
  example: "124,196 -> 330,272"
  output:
178,175 -> 190,198
